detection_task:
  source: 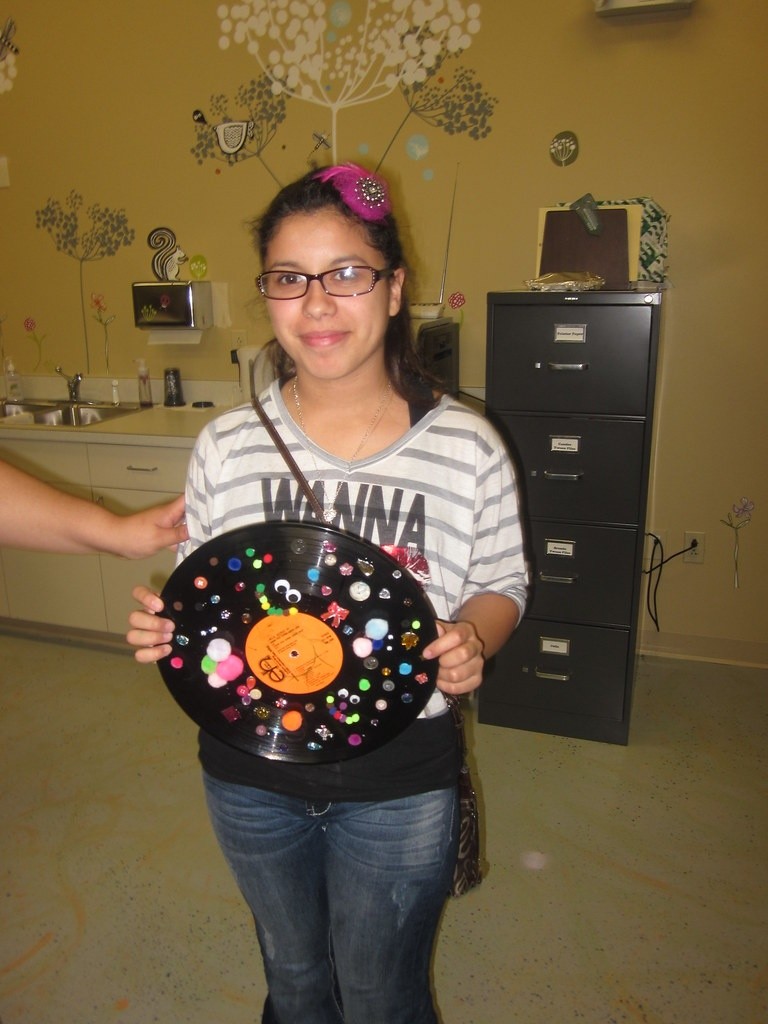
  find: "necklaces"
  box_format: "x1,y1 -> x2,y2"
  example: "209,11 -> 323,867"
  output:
292,372 -> 392,523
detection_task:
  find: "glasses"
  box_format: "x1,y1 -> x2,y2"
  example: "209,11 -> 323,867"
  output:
255,265 -> 398,300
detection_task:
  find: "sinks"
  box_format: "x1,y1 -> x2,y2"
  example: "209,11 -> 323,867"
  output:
0,402 -> 56,418
2,406 -> 138,427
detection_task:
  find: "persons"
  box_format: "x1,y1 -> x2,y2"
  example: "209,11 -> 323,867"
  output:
0,459 -> 190,557
126,161 -> 532,1024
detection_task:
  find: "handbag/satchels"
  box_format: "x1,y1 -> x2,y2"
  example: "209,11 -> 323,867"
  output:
446,762 -> 482,896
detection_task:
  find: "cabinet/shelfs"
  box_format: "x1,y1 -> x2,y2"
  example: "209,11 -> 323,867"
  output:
0,440 -> 190,655
478,292 -> 661,745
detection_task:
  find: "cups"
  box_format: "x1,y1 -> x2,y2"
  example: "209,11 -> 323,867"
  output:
164,368 -> 182,405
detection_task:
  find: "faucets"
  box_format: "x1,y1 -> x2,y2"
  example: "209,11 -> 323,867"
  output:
55,366 -> 83,402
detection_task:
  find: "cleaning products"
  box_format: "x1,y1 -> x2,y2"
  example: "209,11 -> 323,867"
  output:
135,358 -> 153,408
5,356 -> 25,404
